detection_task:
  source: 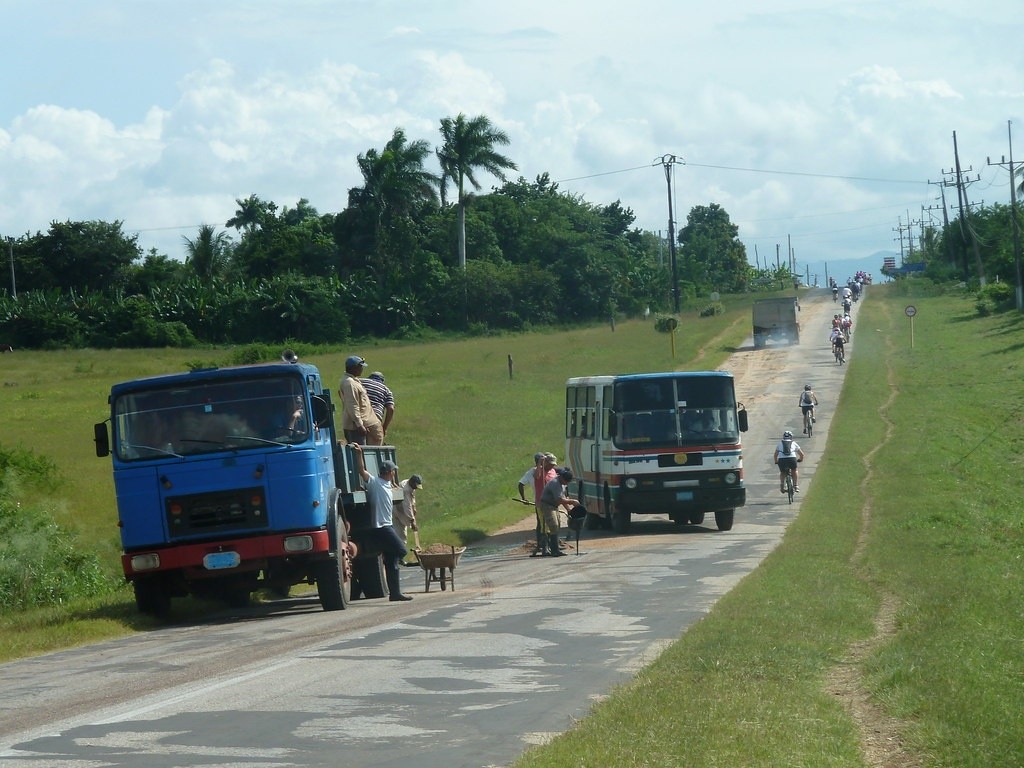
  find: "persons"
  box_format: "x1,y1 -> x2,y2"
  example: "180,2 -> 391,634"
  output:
799,385 -> 818,433
353,441 -> 414,602
518,451 -> 558,557
829,270 -> 873,363
774,431 -> 804,493
338,355 -> 395,446
278,391 -> 318,440
689,410 -> 718,433
392,474 -> 423,566
570,410 -> 595,439
539,471 -> 581,557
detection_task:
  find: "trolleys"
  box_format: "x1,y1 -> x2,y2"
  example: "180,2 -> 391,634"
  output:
410,545 -> 467,592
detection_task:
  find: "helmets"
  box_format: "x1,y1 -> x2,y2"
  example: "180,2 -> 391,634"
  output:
784,431 -> 792,438
805,385 -> 811,390
833,313 -> 848,335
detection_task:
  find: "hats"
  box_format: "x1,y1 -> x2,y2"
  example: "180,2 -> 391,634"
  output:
547,455 -> 557,466
411,474 -> 423,490
535,453 -> 547,460
371,372 -> 384,383
380,460 -> 398,473
346,356 -> 368,372
563,472 -> 573,481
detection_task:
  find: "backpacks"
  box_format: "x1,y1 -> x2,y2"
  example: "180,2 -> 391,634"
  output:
778,440 -> 795,455
802,391 -> 813,404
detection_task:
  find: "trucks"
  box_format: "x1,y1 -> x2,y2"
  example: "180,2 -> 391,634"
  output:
94,350 -> 408,612
752,296 -> 802,348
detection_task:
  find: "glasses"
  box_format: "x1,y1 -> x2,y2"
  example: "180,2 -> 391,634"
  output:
359,357 -> 365,365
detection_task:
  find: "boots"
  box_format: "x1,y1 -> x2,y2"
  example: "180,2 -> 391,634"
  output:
541,533 -> 551,556
550,534 -> 568,557
364,534 -> 394,556
386,568 -> 413,601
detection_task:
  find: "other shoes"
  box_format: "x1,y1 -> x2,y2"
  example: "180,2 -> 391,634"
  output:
803,428 -> 806,434
812,417 -> 816,423
842,359 -> 846,363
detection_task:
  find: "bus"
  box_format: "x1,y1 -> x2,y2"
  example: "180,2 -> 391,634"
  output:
565,372 -> 750,535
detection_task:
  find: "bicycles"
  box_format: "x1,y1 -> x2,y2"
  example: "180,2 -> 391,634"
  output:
799,404 -> 818,438
832,289 -> 862,366
774,459 -> 804,505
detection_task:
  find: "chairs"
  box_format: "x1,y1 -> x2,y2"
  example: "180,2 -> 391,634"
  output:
252,394 -> 295,436
128,414 -> 183,452
689,413 -> 715,427
